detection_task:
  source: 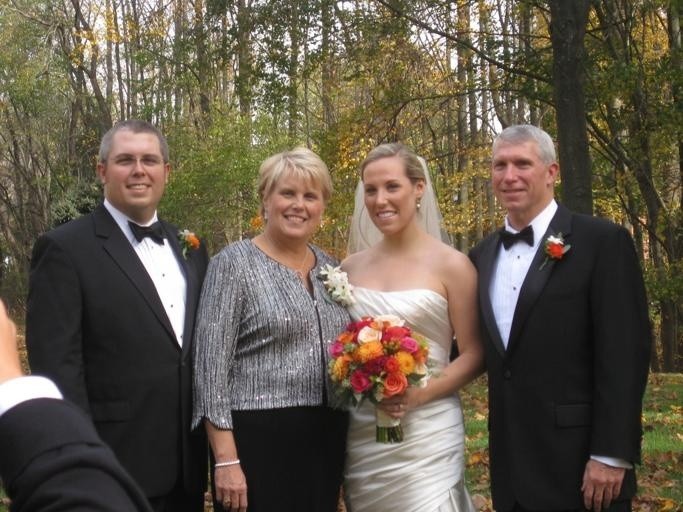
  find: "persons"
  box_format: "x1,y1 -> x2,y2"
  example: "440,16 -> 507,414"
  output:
21,117 -> 210,511
0,298 -> 158,512
187,146 -> 351,512
447,124 -> 653,512
335,141 -> 487,511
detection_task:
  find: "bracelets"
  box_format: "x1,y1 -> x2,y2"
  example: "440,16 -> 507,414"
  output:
213,459 -> 241,468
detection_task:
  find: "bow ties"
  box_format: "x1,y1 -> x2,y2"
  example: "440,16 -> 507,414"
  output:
499,226 -> 533,250
128,221 -> 164,245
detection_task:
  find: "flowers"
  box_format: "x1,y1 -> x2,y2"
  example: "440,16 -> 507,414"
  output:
175,225 -> 203,260
536,228 -> 575,274
328,309 -> 439,447
315,259 -> 353,310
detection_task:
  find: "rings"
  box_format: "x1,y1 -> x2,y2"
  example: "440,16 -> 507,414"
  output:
398,404 -> 404,410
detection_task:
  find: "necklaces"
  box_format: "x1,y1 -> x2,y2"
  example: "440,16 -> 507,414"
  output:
296,247 -> 310,272
